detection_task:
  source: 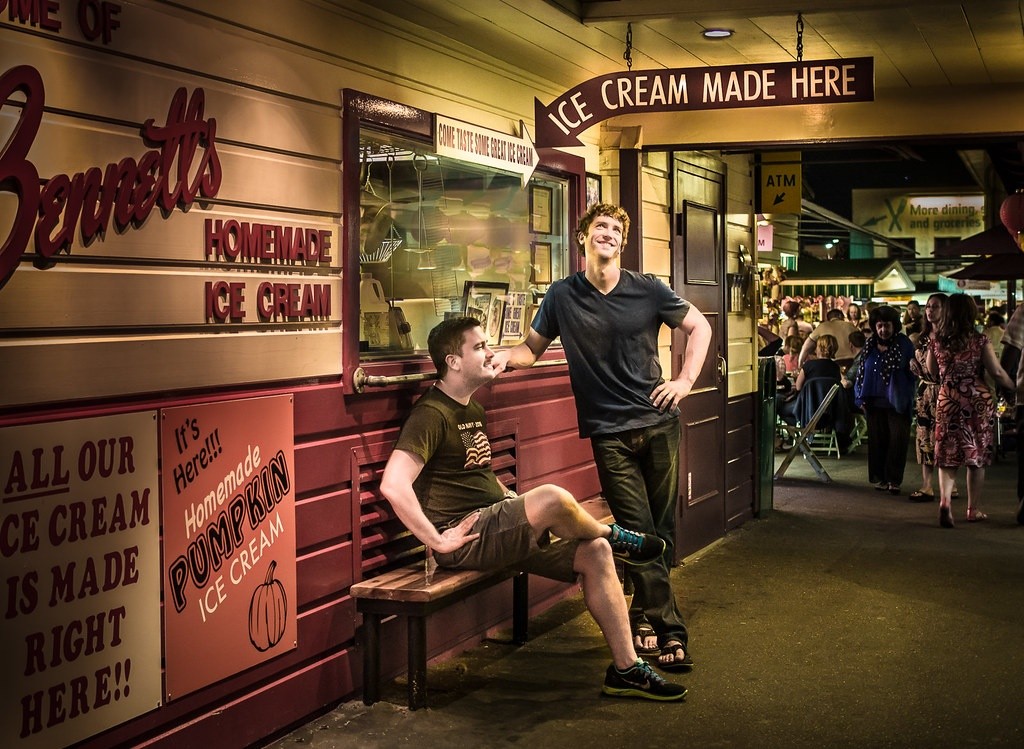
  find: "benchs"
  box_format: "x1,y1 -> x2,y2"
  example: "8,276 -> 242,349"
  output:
348,498 -> 636,712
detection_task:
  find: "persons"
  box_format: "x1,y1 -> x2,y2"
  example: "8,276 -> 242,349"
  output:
492,205 -> 712,667
764,294 -> 1024,528
380,318 -> 688,701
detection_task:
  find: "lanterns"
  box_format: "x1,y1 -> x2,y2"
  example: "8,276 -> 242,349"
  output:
1000,188 -> 1024,251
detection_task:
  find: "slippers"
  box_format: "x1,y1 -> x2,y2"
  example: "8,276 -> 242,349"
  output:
909,490 -> 935,502
951,488 -> 959,499
655,645 -> 694,668
632,627 -> 661,656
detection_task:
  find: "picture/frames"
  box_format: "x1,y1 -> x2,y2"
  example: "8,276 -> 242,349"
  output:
460,184 -> 554,348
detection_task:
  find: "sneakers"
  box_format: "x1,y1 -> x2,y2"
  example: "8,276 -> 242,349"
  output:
607,523 -> 666,566
602,657 -> 688,700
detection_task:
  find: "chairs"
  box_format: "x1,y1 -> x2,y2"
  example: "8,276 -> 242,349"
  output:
773,377 -> 869,484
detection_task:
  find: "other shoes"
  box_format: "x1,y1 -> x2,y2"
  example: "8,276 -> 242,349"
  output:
967,508 -> 987,521
775,442 -> 792,453
888,482 -> 901,494
939,498 -> 955,528
1016,496 -> 1024,524
873,482 -> 888,490
